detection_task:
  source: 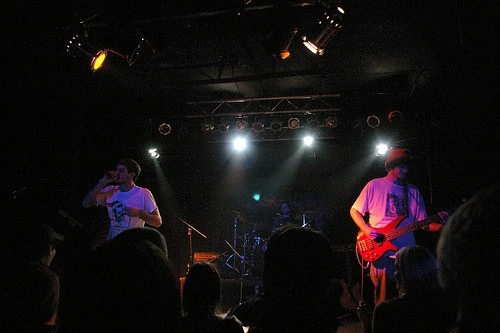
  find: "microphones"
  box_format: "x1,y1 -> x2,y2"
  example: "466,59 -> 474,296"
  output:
106,172 -> 120,178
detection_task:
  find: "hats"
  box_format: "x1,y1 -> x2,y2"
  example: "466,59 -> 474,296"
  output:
385,148 -> 412,171
268,224 -> 333,272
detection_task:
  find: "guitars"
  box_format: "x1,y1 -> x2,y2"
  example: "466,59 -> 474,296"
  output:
355,205 -> 466,263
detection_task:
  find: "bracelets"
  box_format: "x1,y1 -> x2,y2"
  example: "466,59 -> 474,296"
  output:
95,181 -> 106,191
139,210 -> 148,221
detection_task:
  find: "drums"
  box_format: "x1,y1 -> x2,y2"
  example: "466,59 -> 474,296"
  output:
234,232 -> 266,262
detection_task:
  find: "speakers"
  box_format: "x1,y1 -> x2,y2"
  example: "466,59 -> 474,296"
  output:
192,251 -> 224,267
224,298 -> 254,325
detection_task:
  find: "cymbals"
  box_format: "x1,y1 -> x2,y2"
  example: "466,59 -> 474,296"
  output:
226,208 -> 249,223
296,209 -> 314,214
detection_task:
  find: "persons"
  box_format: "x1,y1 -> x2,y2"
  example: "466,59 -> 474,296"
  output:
350,149 -> 450,305
436,182 -> 500,333
83,158 -> 162,241
273,201 -> 295,232
371,245 -> 457,333
0,224 -> 257,333
234,225 -> 344,333
